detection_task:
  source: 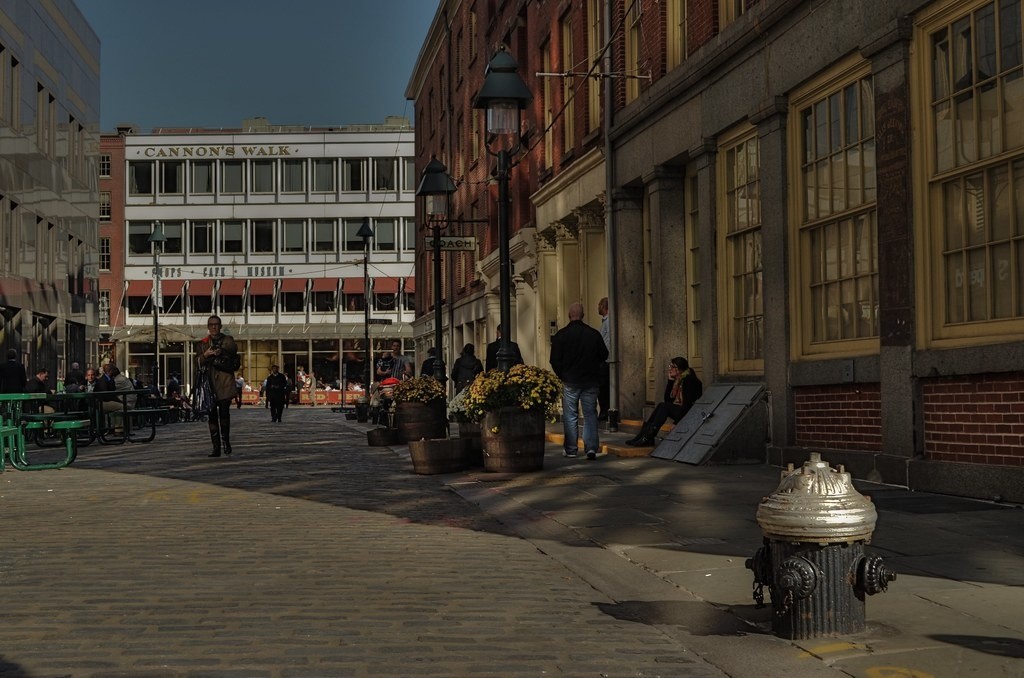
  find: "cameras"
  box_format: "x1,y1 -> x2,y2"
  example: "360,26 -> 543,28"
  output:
211,339 -> 219,347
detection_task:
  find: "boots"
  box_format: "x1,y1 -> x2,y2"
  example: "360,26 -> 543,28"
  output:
625,420 -> 660,447
208,420 -> 222,457
220,414 -> 232,455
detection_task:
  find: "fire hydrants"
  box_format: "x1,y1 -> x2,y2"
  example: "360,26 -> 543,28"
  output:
745,451 -> 897,642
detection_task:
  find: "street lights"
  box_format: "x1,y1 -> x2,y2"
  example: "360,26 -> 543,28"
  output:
415,155 -> 456,384
356,223 -> 375,397
472,44 -> 533,375
148,221 -> 167,393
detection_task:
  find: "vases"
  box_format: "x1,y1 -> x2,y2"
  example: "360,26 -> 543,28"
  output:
479,405 -> 547,474
366,428 -> 399,447
407,437 -> 470,475
459,422 -> 484,467
395,399 -> 446,444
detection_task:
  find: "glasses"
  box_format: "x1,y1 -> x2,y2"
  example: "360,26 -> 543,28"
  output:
669,364 -> 678,369
208,322 -> 220,327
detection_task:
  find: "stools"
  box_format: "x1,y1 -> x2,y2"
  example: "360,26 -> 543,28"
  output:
0,405 -> 174,439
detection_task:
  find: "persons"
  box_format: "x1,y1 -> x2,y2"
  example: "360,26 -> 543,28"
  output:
283,373 -> 292,409
451,343 -> 484,397
261,373 -> 271,409
625,357 -> 702,447
486,324 -> 524,377
64,362 -> 181,436
549,302 -> 609,460
598,298 -> 610,422
296,365 -> 366,407
235,373 -> 244,409
420,347 -> 448,392
370,338 -> 412,424
0,348 -> 27,412
265,365 -> 287,423
23,368 -> 55,439
195,315 -> 240,457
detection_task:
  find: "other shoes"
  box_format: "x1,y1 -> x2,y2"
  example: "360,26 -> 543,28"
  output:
587,450 -> 596,459
597,415 -> 608,421
114,432 -> 126,437
44,433 -> 47,439
562,450 -> 578,458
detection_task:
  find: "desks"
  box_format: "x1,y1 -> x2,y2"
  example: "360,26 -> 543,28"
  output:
33,389 -> 157,447
0,393 -> 78,473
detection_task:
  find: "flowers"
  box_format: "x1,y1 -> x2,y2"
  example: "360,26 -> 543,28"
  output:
390,373 -> 446,402
447,364 -> 567,433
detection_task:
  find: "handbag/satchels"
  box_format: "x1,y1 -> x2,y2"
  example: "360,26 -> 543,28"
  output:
193,356 -> 217,416
221,354 -> 241,372
